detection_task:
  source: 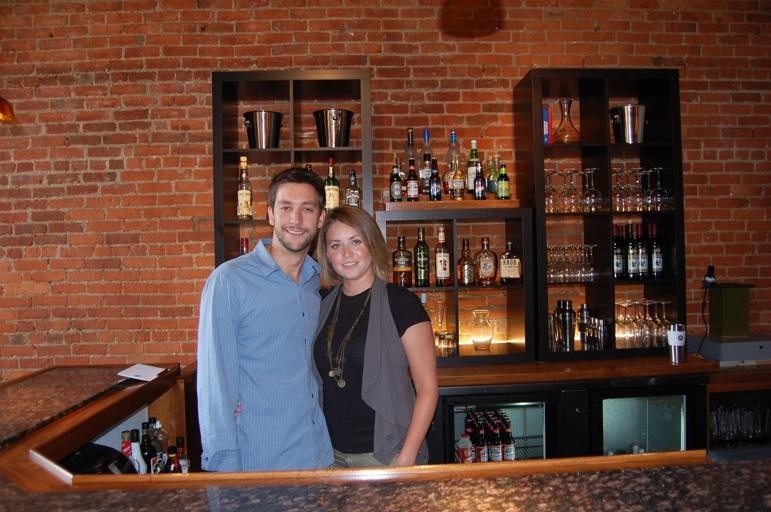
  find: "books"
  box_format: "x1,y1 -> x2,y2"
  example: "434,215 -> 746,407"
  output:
116,363 -> 167,382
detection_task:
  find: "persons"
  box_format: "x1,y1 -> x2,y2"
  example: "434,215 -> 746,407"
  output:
233,205 -> 440,469
196,167 -> 336,472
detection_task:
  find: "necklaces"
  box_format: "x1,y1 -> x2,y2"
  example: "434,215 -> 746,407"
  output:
326,284 -> 372,389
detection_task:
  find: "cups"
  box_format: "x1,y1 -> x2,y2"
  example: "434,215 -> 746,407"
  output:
668,323 -> 690,366
611,105 -> 650,145
711,402 -> 770,442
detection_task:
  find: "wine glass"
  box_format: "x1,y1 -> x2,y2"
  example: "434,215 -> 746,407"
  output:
434,301 -> 447,330
548,243 -> 601,284
544,166 -> 674,215
613,296 -> 673,351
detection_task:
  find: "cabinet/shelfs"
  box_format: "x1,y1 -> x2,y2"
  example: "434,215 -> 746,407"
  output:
510,63 -> 690,363
373,202 -> 540,371
211,66 -> 376,277
705,363 -> 771,461
411,367 -> 711,465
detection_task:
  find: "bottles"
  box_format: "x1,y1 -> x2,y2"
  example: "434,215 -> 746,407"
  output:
458,408 -> 518,462
121,417 -> 190,475
471,309 -> 495,350
615,220 -> 663,280
391,226 -> 522,288
237,156 -> 253,221
552,97 -> 583,142
306,158 -> 362,210
420,292 -> 432,319
391,127 -> 512,202
551,298 -> 608,351
436,329 -> 457,349
239,237 -> 249,254
